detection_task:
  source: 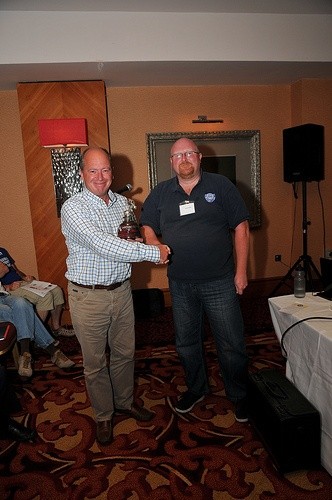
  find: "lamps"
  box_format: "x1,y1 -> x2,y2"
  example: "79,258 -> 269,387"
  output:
38,118 -> 89,150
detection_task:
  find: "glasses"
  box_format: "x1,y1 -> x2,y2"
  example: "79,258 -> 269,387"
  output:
84,167 -> 114,175
170,151 -> 200,158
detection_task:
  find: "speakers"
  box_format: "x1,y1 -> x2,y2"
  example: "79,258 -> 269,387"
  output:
282,122 -> 326,185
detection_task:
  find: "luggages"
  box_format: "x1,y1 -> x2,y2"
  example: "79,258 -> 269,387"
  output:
247,366 -> 323,474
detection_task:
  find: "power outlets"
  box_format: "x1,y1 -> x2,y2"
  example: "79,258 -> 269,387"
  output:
275,254 -> 282,262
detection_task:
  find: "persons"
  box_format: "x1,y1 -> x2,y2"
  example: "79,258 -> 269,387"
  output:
61,147 -> 169,446
0,247 -> 77,441
141,138 -> 256,422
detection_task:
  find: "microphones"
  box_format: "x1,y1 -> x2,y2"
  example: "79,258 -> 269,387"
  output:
117,183 -> 132,195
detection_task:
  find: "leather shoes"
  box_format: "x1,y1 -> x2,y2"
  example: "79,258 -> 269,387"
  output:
96,420 -> 113,446
115,401 -> 155,422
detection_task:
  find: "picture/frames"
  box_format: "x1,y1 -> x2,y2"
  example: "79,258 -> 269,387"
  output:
146,130 -> 262,235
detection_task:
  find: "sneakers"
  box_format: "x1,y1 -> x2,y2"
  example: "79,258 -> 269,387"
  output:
175,392 -> 205,414
17,352 -> 33,377
51,351 -> 76,369
233,401 -> 249,423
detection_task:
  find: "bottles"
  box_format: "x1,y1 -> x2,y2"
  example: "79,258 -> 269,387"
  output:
294,264 -> 305,297
117,211 -> 142,242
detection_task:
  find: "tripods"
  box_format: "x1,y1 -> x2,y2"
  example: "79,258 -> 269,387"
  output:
265,181 -> 327,298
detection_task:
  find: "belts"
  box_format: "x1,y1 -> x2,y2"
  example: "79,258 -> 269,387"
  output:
66,277 -> 131,291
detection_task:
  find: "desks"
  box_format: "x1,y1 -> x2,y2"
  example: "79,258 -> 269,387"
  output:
0,322 -> 38,445
268,291 -> 332,475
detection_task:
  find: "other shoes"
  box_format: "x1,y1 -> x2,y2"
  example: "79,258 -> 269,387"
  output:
50,325 -> 76,337
